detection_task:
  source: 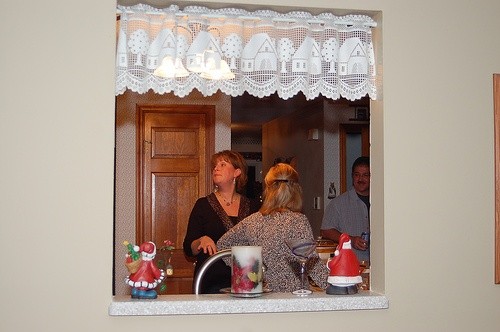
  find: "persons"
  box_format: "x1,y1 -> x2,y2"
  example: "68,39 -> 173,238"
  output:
321,155 -> 370,268
215,162 -> 331,292
183,148 -> 257,295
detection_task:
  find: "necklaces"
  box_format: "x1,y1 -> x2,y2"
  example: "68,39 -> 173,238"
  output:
218,190 -> 239,207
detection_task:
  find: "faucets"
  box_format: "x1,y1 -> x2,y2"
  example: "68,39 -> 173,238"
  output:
192,248 -> 268,295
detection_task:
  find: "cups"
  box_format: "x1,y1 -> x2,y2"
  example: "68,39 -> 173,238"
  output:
360,260 -> 370,291
231,246 -> 264,299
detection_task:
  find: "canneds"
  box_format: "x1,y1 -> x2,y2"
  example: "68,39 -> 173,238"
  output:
362,232 -> 369,248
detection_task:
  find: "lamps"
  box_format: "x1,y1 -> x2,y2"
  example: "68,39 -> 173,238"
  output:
158,240 -> 175,276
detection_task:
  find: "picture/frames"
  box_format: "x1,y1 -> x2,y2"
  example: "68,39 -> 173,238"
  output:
355,107 -> 368,119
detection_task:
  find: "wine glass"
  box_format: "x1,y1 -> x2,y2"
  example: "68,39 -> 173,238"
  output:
284,237 -> 322,296
